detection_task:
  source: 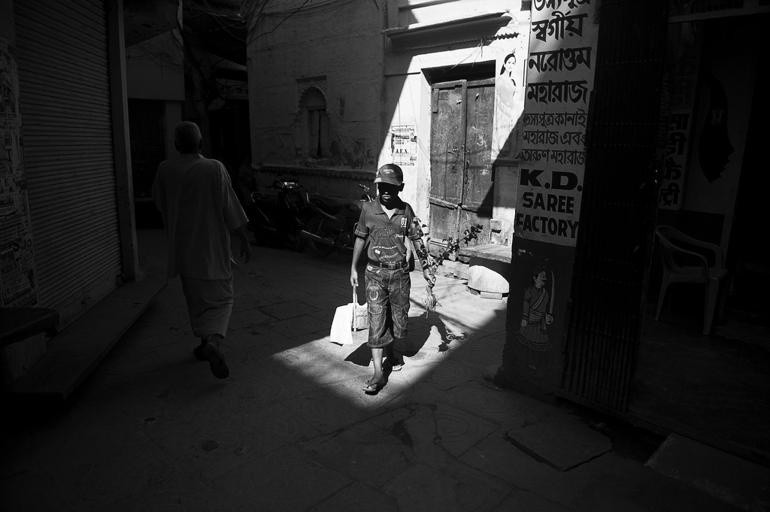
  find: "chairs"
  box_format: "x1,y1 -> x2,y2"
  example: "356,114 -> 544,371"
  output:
653,224 -> 728,337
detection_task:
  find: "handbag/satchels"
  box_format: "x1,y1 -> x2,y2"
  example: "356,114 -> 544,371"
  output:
352,284 -> 368,331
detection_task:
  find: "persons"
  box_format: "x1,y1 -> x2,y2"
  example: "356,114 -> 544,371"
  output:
350,162 -> 436,394
153,121 -> 252,378
517,267 -> 555,372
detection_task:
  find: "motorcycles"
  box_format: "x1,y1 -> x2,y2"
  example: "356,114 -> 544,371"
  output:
275,177 -> 375,258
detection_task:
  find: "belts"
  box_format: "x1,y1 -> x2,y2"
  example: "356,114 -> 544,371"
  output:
367,258 -> 406,270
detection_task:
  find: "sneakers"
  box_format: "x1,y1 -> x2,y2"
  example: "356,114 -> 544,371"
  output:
194,344 -> 229,378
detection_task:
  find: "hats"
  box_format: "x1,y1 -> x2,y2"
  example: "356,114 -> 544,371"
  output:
373,164 -> 403,186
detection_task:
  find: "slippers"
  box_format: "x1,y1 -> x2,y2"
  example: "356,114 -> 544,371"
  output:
362,376 -> 387,392
